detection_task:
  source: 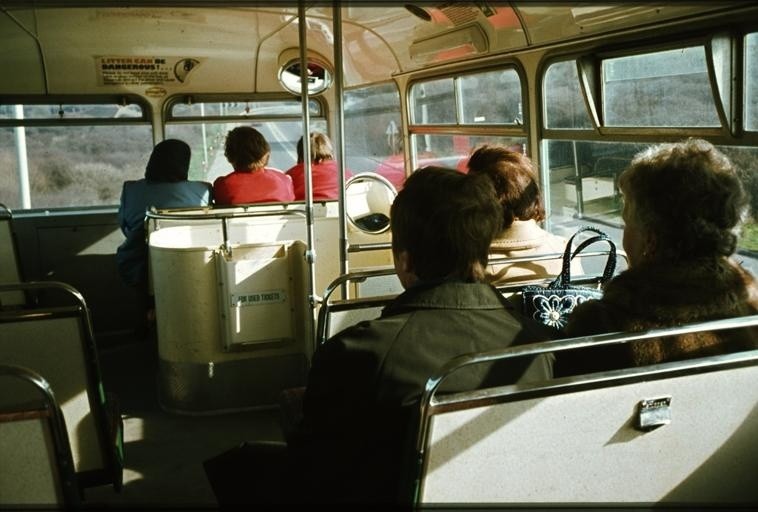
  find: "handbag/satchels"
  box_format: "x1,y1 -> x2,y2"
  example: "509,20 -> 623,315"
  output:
520,225 -> 616,329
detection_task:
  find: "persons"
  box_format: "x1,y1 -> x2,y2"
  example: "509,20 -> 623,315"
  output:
213,125 -> 296,204
200,163 -> 564,504
565,134 -> 758,372
284,131 -> 354,201
466,141 -> 586,286
114,137 -> 214,287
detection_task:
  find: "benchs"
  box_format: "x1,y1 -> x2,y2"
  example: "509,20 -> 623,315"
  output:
300,244 -> 635,354
399,303 -> 756,509
3,278 -> 130,495
142,192 -> 344,306
1,356 -> 88,511
1,199 -> 29,308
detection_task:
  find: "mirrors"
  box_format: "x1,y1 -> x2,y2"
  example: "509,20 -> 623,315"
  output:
343,173 -> 400,236
278,57 -> 334,99
